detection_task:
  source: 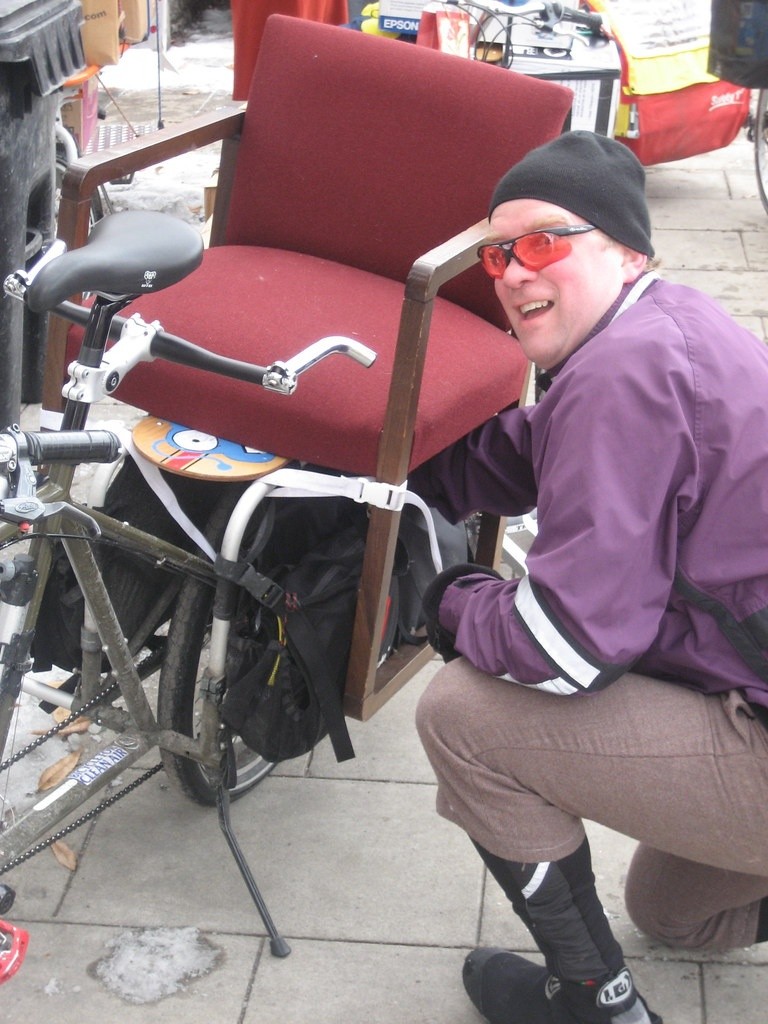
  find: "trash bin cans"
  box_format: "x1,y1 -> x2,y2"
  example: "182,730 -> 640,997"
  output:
0,0 -> 86,478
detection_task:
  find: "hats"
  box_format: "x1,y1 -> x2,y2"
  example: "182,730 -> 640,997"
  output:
488,130 -> 655,260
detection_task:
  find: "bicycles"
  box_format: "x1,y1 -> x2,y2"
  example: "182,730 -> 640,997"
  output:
0,208 -> 498,959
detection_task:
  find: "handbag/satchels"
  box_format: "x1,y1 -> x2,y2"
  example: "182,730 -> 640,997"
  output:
28,451 -> 239,677
219,502 -> 474,761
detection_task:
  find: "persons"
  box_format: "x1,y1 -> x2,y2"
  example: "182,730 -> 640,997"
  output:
403,128 -> 768,1024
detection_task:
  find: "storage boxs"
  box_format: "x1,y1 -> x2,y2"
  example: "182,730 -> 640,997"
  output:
81,0 -> 119,66
119,0 -> 156,44
61,73 -> 98,158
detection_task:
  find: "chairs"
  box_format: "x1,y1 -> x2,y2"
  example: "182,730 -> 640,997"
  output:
42,14 -> 575,722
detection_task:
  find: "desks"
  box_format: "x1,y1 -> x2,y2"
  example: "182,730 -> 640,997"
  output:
491,30 -> 624,136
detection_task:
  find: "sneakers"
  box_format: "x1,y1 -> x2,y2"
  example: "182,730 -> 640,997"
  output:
461,946 -> 664,1024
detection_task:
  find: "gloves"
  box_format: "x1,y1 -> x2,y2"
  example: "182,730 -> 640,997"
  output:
423,562 -> 507,664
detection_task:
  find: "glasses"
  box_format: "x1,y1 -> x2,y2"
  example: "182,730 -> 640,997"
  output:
477,224 -> 597,279
